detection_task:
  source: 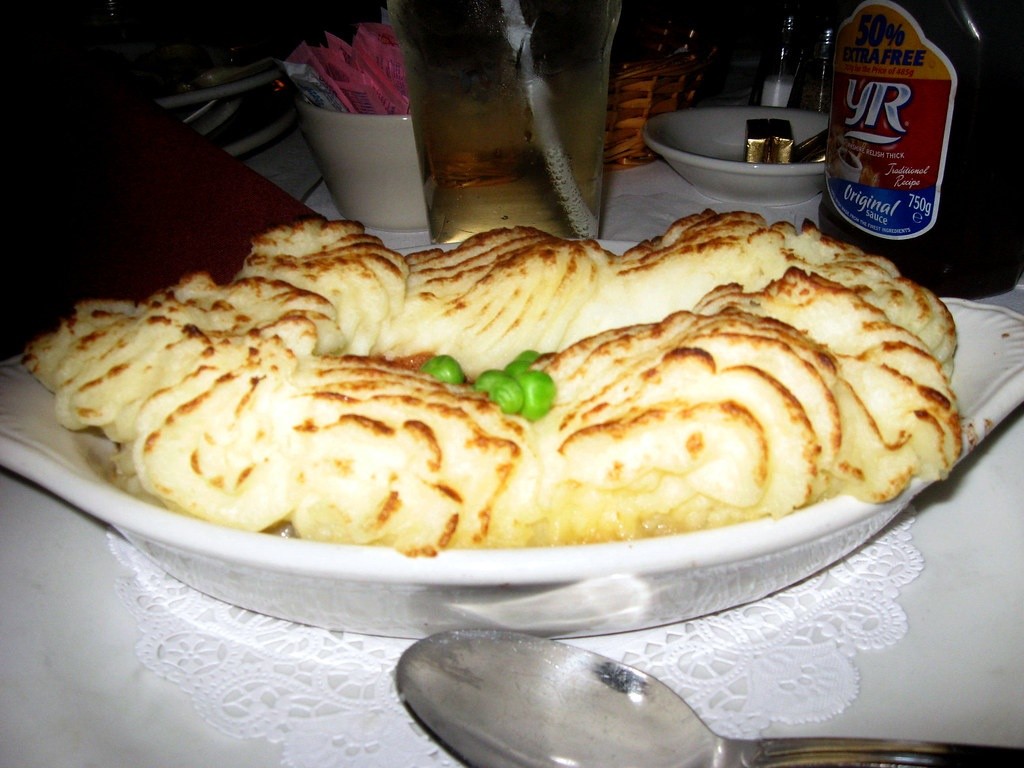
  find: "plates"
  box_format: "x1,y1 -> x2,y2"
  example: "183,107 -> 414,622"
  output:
0,238 -> 1024,640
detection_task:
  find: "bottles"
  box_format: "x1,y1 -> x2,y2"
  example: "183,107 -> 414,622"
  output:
384,0 -> 622,237
760,0 -> 803,108
819,0 -> 1024,300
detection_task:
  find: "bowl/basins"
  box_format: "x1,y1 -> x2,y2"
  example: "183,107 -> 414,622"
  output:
641,107 -> 829,207
295,96 -> 428,232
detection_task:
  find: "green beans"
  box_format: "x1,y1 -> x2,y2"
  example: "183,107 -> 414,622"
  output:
418,343 -> 556,420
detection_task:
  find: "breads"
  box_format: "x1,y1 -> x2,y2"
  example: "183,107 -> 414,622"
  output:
16,212 -> 964,563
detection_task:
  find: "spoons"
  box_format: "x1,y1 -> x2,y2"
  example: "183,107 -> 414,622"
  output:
394,627 -> 1024,768
